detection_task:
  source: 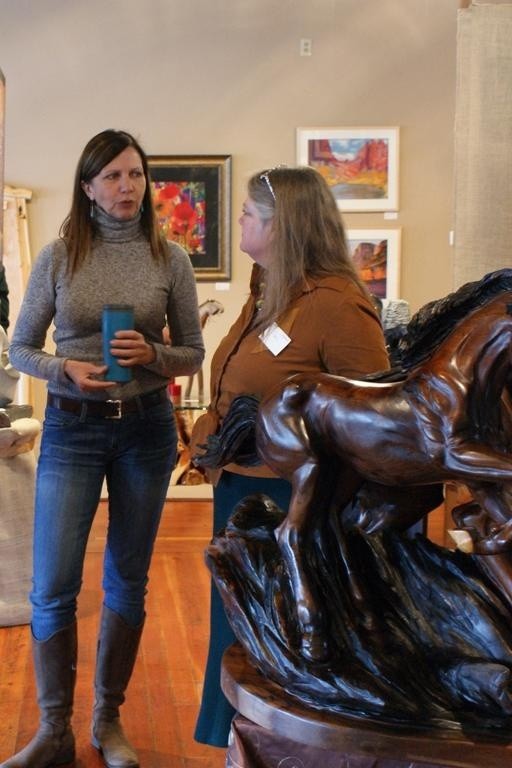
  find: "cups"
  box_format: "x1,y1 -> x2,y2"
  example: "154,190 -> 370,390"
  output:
101,304 -> 137,383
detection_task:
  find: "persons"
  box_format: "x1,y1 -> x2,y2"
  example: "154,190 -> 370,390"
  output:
2,128 -> 204,766
193,166 -> 391,746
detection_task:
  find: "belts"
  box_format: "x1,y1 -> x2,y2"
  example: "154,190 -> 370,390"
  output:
46,393 -> 166,420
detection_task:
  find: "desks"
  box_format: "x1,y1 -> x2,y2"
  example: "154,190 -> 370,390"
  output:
168,393 -> 214,486
213,630 -> 512,767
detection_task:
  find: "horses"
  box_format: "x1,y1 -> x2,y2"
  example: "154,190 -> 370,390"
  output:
190,268 -> 512,662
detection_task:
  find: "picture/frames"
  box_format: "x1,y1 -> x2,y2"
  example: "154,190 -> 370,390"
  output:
292,123 -> 401,217
339,225 -> 401,327
145,153 -> 234,286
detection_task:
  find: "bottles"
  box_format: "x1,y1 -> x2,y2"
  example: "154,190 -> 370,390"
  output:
169,384 -> 183,404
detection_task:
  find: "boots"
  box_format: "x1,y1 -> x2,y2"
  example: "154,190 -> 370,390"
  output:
89,602 -> 146,767
1,619 -> 79,767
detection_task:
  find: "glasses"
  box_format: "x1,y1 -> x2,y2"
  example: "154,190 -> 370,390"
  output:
260,165 -> 285,199
